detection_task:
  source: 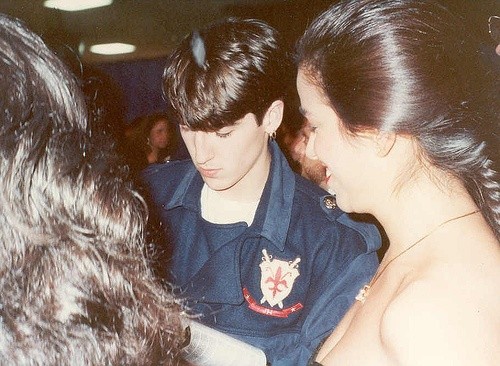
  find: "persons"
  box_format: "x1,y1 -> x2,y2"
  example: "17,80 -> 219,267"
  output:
296,0 -> 500,366
136,16 -> 382,366
130,113 -> 171,170
0,14 -> 182,366
281,85 -> 327,183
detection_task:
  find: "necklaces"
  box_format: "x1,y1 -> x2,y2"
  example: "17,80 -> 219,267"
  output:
356,208 -> 483,300
